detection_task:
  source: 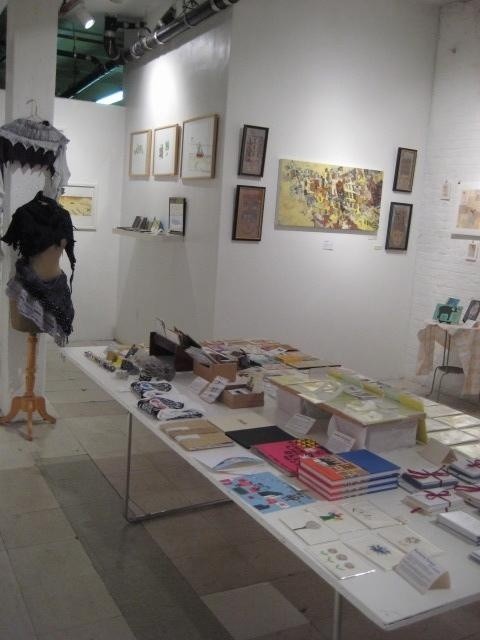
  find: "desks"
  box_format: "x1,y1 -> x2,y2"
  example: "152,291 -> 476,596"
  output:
60,336 -> 480,640
416,323 -> 479,405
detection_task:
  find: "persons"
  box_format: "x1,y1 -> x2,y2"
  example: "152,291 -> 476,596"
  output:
2,190 -> 76,334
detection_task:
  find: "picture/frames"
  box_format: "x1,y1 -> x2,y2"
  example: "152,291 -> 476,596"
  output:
392,147 -> 418,194
231,185 -> 266,241
239,124 -> 270,178
385,202 -> 413,252
449,182 -> 480,239
60,184 -> 98,233
129,113 -> 220,179
168,196 -> 185,237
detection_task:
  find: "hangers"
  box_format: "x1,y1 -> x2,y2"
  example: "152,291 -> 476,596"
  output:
0,98 -> 71,149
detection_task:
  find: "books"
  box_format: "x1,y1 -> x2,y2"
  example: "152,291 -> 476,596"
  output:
262,344 -> 343,372
156,418 -> 401,502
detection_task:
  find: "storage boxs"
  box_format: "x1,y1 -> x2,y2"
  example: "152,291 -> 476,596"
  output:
218,383 -> 265,409
191,351 -> 239,383
